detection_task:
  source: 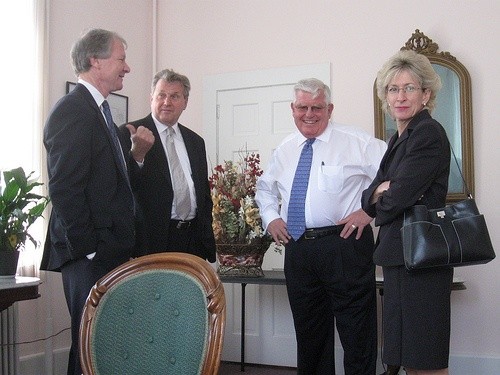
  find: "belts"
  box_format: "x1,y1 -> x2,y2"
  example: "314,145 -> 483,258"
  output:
171,218 -> 193,232
303,225 -> 344,239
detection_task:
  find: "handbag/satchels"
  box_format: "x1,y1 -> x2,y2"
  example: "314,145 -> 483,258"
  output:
400,194 -> 496,271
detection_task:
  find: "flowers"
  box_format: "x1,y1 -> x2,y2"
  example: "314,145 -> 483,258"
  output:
209,140 -> 276,244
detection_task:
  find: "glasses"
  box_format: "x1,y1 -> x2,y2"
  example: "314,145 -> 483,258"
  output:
386,85 -> 423,95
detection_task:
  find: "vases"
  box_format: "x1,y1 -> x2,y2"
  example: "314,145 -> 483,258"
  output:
215,235 -> 270,277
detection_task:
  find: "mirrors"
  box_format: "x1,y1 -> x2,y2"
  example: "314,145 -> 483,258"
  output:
374,29 -> 473,202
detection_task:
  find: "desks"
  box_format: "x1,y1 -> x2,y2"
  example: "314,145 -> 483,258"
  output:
219,270 -> 465,372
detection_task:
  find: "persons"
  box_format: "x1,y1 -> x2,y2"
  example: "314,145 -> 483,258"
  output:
256,78 -> 388,375
119,70 -> 216,263
361,53 -> 453,375
40,28 -> 136,375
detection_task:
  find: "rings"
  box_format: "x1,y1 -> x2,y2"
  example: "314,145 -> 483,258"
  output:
353,225 -> 356,228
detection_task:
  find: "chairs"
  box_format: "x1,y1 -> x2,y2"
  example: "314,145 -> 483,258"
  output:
77,252 -> 226,375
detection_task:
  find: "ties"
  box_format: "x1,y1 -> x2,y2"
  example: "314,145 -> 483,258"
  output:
166,127 -> 190,221
102,100 -> 130,187
287,138 -> 315,241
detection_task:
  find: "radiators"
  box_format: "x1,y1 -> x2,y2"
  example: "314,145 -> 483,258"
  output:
0,300 -> 20,375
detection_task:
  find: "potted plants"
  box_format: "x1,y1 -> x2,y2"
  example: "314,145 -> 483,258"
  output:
0,166 -> 53,278
